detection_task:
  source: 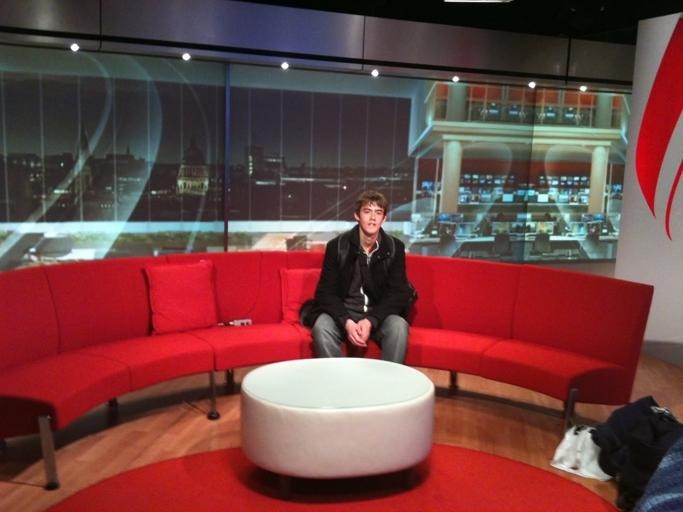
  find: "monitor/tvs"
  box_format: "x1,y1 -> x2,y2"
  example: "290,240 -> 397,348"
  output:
437,213 -> 610,237
422,173 -> 621,204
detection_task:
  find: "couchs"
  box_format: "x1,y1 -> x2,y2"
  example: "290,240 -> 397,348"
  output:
0,249 -> 654,490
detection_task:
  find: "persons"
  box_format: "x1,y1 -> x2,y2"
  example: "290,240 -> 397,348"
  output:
307,189 -> 412,364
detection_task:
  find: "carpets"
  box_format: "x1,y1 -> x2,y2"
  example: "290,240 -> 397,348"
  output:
42,442 -> 624,512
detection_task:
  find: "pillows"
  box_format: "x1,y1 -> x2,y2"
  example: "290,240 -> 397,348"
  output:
280,268 -> 322,325
142,259 -> 221,336
